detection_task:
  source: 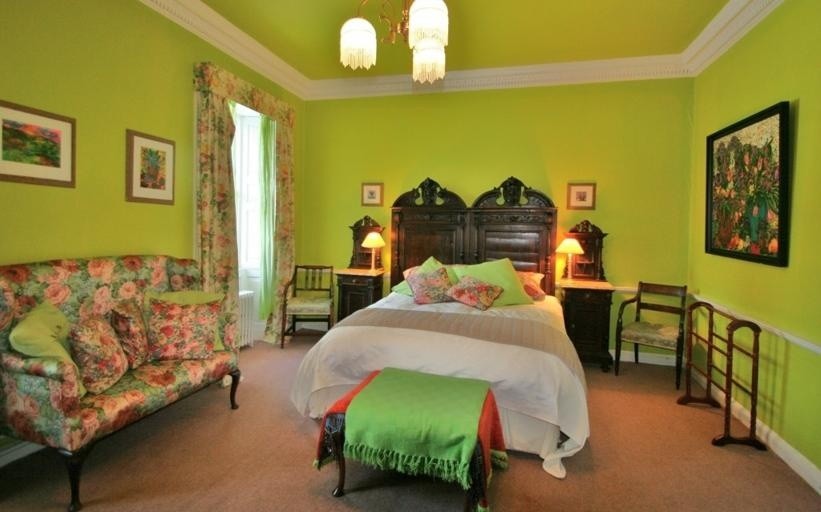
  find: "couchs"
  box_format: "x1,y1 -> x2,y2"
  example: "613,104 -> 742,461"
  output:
0,254 -> 241,511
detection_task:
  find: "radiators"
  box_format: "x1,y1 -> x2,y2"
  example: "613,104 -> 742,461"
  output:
238,289 -> 257,350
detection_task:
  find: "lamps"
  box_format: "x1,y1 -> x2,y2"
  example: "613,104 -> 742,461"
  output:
360,231 -> 386,274
339,0 -> 449,86
553,237 -> 585,283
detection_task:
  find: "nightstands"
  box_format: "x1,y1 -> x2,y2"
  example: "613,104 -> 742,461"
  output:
558,219 -> 615,373
332,215 -> 387,323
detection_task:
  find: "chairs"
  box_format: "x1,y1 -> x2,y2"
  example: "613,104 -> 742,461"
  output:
280,264 -> 334,350
614,280 -> 688,391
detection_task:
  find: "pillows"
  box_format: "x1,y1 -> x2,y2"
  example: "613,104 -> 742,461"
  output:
391,256 -> 548,312
7,289 -> 230,401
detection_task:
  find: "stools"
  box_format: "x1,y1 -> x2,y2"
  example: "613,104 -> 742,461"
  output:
321,366 -> 495,512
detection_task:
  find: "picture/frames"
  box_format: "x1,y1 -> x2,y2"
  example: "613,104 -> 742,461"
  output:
566,181 -> 597,211
360,182 -> 384,208
0,98 -> 175,206
703,99 -> 792,269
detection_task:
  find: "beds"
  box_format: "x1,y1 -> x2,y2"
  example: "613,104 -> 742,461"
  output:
303,176 -> 563,463
